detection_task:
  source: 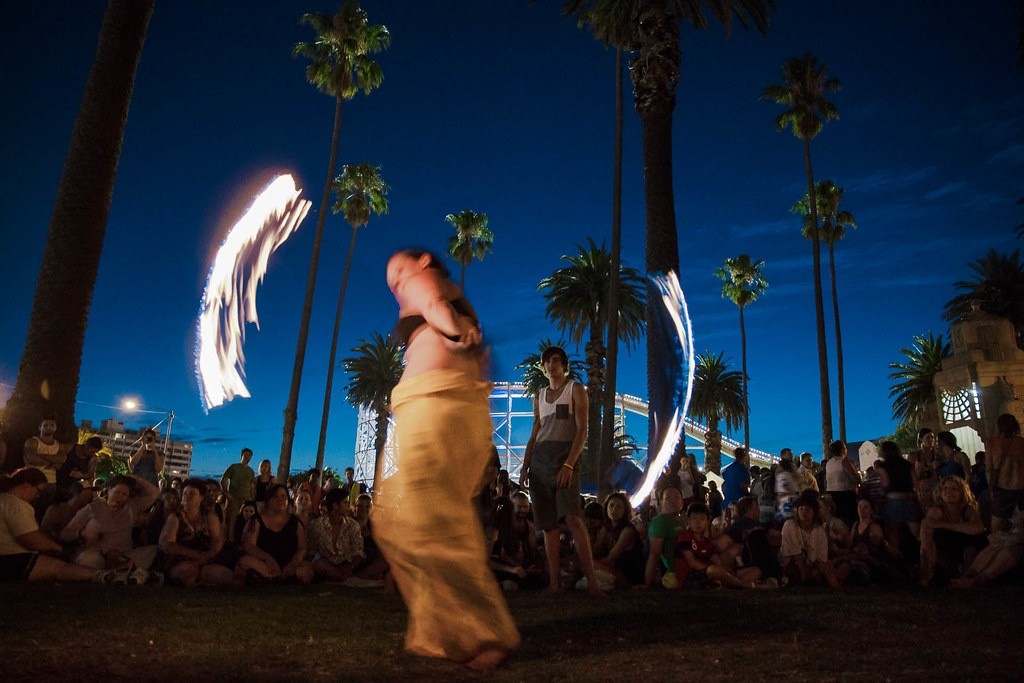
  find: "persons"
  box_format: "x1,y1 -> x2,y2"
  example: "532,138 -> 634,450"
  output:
0,414 -> 1023,601
366,247 -> 523,671
519,345 -> 608,600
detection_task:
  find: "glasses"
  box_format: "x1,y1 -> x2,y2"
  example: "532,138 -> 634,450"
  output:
35,485 -> 43,494
